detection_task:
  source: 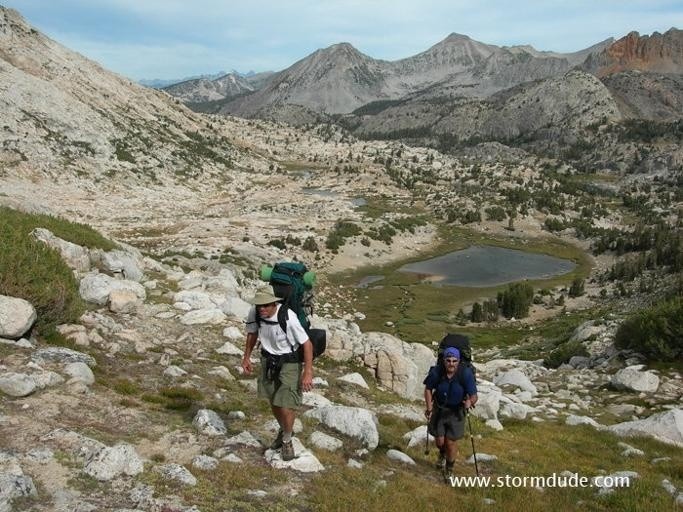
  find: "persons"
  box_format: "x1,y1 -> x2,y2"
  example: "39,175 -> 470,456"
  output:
422,347 -> 478,484
240,287 -> 311,461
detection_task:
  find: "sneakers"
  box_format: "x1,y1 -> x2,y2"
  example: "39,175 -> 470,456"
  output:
436,454 -> 444,469
271,428 -> 295,450
444,459 -> 455,483
281,438 -> 295,461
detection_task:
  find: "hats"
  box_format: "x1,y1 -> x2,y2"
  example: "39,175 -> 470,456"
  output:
249,285 -> 284,305
443,347 -> 460,360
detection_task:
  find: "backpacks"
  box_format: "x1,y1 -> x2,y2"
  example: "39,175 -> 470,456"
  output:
242,262 -> 317,381
432,334 -> 476,415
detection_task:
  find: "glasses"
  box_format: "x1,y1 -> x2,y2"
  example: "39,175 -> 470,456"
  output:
446,359 -> 458,363
